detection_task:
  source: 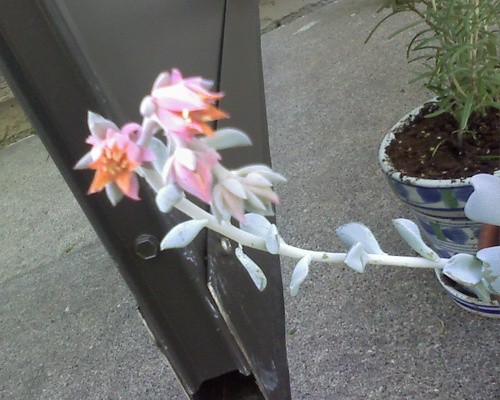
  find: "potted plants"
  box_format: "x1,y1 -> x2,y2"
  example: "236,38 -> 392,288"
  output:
371,0 -> 500,319
